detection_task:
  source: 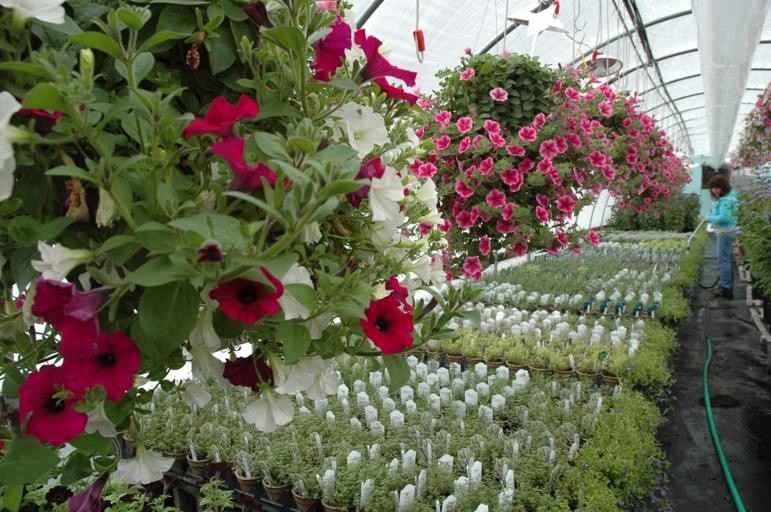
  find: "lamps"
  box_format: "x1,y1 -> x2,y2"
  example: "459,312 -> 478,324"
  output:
575,1 -> 623,77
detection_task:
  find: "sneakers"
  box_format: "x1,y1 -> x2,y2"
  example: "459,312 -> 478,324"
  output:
713,289 -> 731,297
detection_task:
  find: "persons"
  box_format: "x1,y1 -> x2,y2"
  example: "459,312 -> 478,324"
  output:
703,175 -> 739,297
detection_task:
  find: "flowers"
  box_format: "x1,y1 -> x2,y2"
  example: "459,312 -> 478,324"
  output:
406,50 -> 690,279
728,84 -> 771,173
0,0 -> 481,498
